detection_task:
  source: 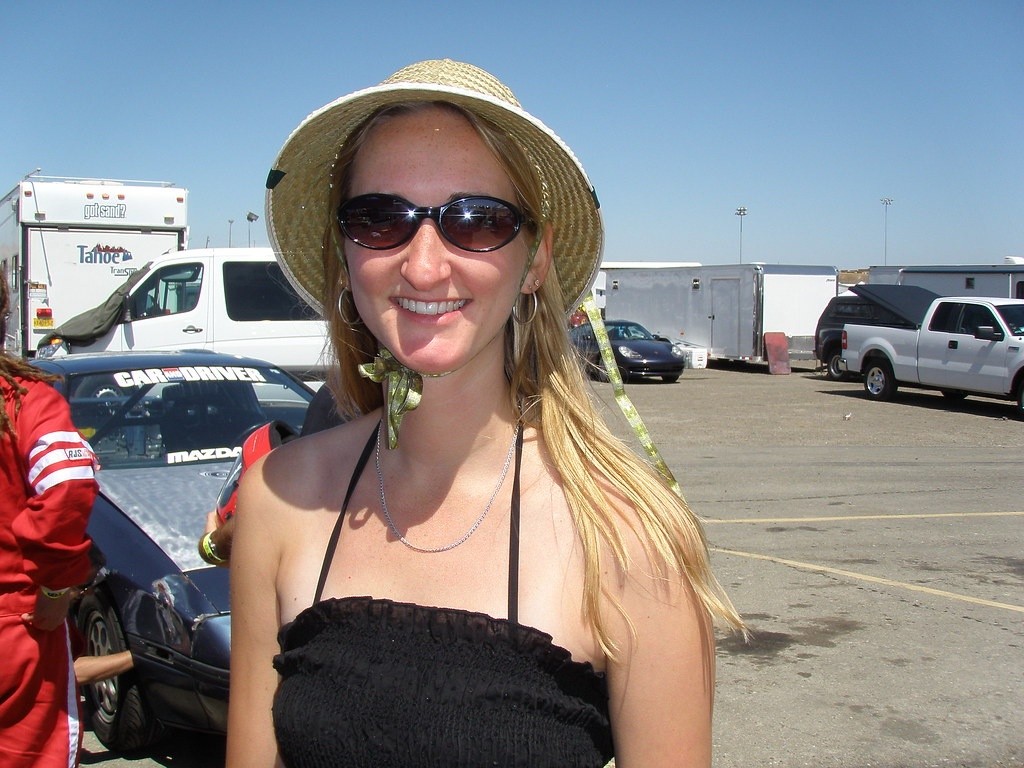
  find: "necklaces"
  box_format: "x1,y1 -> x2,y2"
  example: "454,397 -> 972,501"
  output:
375,417 -> 518,553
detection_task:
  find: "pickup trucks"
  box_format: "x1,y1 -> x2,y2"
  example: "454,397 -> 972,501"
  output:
836,284 -> 1024,417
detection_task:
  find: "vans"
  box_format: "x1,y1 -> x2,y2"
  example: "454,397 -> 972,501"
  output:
34,243 -> 339,423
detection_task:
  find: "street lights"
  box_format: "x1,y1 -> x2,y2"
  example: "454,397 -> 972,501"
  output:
228,218 -> 233,246
245,212 -> 258,245
736,206 -> 749,264
880,196 -> 895,268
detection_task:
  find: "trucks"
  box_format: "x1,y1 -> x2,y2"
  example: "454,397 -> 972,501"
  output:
1,170 -> 192,363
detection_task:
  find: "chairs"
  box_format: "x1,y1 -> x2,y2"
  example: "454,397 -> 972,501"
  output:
163,381 -> 260,455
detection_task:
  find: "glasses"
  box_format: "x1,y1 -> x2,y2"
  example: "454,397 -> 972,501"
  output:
333,193 -> 538,253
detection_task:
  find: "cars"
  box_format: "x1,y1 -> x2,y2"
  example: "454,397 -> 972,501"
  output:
571,309 -> 599,327
18,350 -> 344,746
572,319 -> 685,383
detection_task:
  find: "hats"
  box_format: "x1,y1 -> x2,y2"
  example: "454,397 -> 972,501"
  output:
268,58 -> 604,321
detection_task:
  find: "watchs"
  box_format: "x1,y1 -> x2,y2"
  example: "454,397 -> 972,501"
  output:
41,585 -> 71,599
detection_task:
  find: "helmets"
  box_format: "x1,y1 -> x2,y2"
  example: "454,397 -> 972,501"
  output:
214,419 -> 301,529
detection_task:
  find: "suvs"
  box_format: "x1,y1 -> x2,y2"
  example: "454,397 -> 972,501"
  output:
815,295 -> 922,381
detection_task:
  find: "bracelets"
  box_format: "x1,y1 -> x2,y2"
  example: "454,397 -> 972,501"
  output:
202,531 -> 227,564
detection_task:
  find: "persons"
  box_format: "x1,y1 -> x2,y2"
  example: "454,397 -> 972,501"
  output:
0,268 -> 134,768
198,58 -> 750,768
